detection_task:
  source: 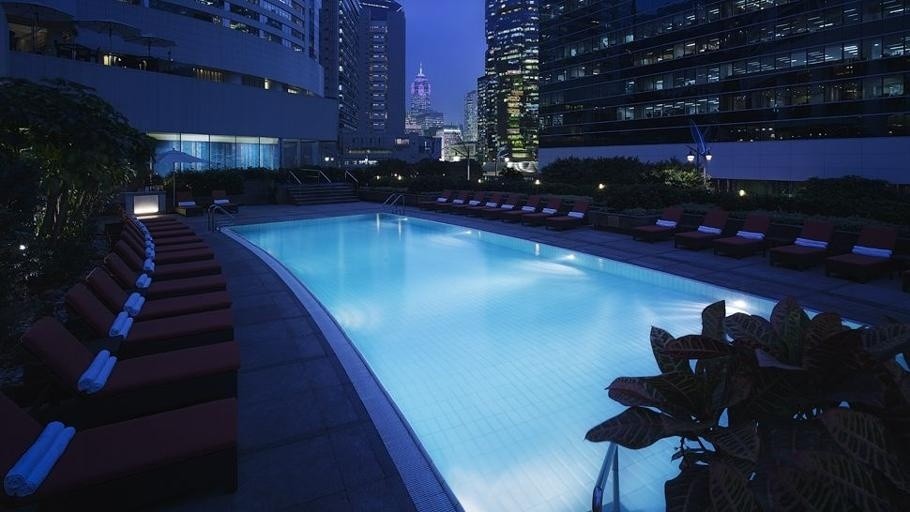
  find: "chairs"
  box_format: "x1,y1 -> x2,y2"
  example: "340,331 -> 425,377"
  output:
632,206 -> 908,292
418,187 -> 591,233
1,189 -> 241,509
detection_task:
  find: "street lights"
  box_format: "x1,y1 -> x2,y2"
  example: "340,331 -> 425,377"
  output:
687,146 -> 714,176
